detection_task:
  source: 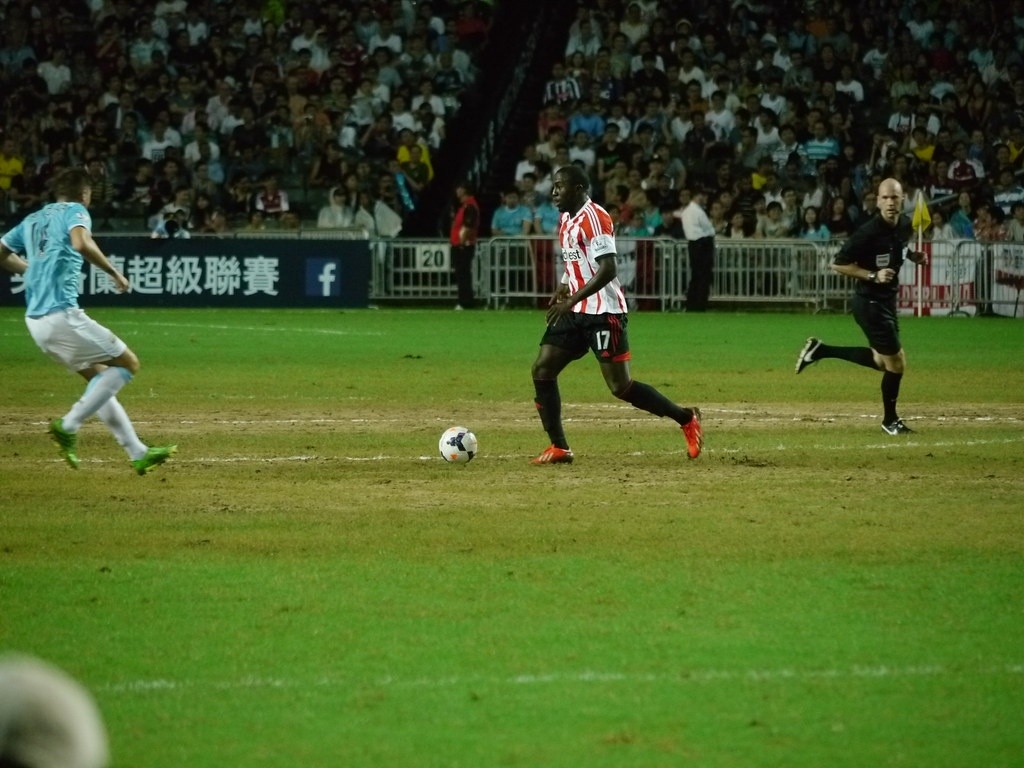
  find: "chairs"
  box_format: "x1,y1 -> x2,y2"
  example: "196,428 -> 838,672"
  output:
86,151 -> 329,233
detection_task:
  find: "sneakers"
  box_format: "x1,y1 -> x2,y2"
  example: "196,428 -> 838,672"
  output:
881,417 -> 909,436
795,336 -> 821,374
131,444 -> 178,476
680,408 -> 703,458
49,418 -> 80,470
531,444 -> 574,464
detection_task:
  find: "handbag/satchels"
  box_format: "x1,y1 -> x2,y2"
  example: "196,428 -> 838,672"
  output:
787,151 -> 800,166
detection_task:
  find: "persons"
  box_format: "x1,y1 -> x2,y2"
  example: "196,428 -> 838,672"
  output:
0,0 -> 490,310
0,168 -> 172,477
531,164 -> 704,465
796,178 -> 929,432
491,0 -> 1024,312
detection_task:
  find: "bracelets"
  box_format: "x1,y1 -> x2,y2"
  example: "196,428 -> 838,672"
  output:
869,270 -> 877,282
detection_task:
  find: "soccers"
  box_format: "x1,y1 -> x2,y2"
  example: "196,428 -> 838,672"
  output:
438,427 -> 478,464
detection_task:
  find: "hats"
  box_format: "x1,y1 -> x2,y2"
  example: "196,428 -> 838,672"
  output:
648,153 -> 663,163
